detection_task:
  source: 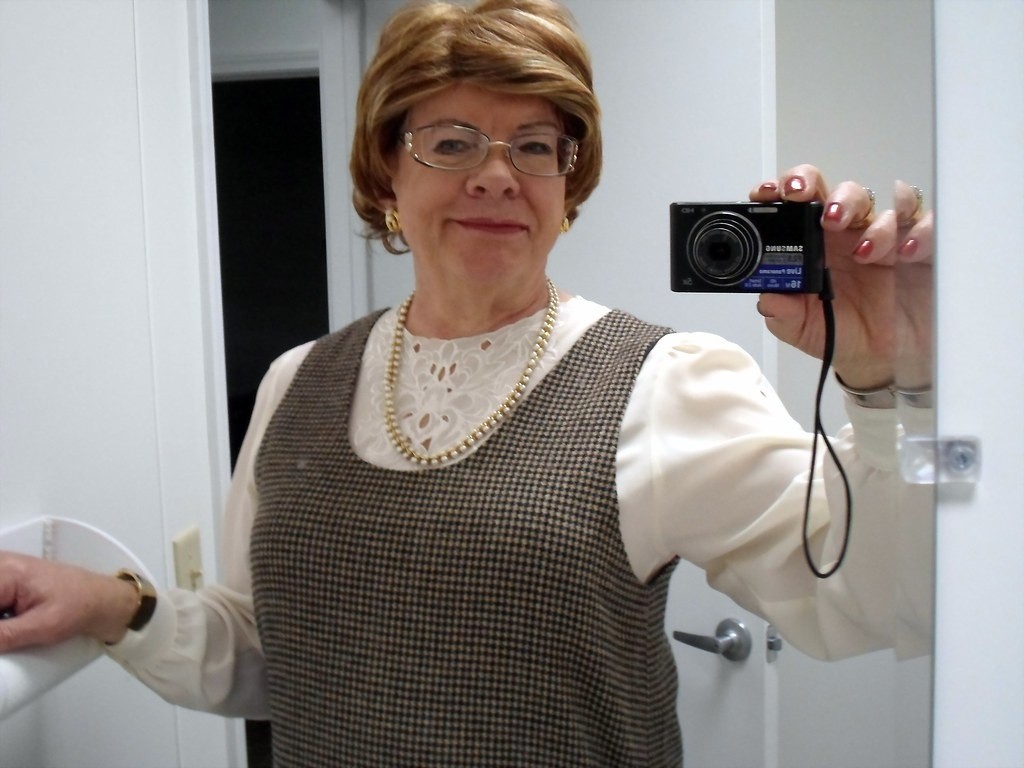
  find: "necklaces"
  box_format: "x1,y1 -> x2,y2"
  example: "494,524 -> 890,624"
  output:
383,273 -> 558,465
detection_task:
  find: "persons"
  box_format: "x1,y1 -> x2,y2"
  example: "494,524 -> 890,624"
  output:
0,0 -> 895,768
895,181 -> 934,663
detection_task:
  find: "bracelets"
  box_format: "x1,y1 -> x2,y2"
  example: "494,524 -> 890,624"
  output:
103,567 -> 157,654
832,370 -> 897,410
895,382 -> 935,409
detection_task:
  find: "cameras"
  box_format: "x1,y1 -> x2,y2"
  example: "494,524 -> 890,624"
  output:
671,199 -> 825,293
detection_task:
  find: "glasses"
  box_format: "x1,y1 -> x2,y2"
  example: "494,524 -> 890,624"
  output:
393,121 -> 579,176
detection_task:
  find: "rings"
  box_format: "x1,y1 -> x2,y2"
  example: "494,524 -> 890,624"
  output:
848,186 -> 877,228
897,185 -> 924,228
756,301 -> 776,320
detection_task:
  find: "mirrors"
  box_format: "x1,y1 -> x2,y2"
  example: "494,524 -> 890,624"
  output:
209,1 -> 938,768
